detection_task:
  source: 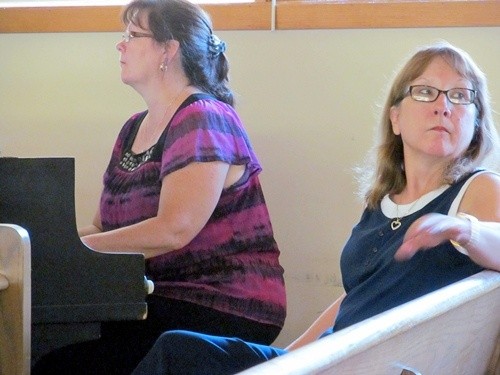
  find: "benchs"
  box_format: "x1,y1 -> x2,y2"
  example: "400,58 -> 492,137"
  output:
235,268 -> 500,374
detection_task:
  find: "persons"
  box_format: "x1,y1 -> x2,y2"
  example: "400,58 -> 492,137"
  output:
31,0 -> 286,375
127,45 -> 500,375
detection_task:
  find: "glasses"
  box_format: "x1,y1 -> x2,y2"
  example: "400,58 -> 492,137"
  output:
394,86 -> 478,112
123,30 -> 156,38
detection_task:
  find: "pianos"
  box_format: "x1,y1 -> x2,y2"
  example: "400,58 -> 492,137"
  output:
0,157 -> 154,367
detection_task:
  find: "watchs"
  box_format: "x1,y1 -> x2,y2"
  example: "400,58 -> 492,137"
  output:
449,213 -> 481,253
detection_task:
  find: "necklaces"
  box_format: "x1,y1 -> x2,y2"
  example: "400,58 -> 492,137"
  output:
390,194 -> 422,230
